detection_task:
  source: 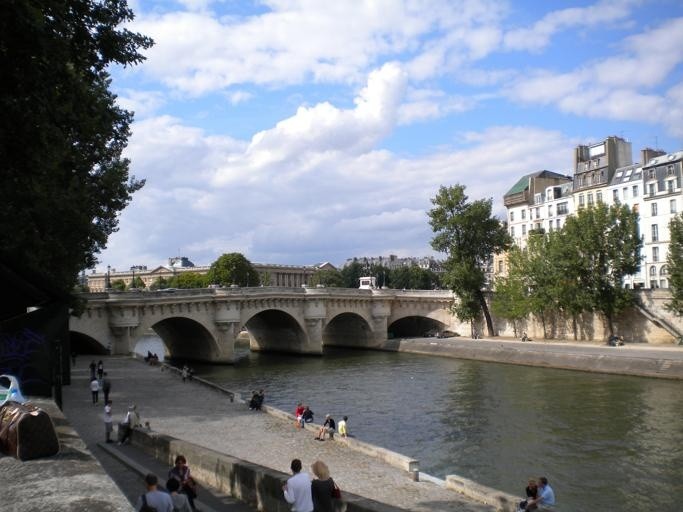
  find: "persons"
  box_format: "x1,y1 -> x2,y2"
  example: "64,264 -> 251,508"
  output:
96,359 -> 103,381
300,405 -> 314,428
337,415 -> 356,439
168,454 -> 198,511
88,359 -> 96,379
187,368 -> 194,381
255,389 -> 264,411
295,401 -> 304,427
518,477 -> 537,511
88,376 -> 100,408
143,349 -> 153,362
102,399 -> 150,446
281,458 -> 313,512
164,476 -> 193,512
311,460 -> 335,512
101,372 -> 111,406
70,349 -> 77,367
525,476 -> 555,512
249,390 -> 258,410
181,366 -> 188,381
148,354 -> 158,366
133,472 -> 174,512
313,413 -> 335,441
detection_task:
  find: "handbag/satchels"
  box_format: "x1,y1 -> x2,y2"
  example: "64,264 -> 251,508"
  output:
1,401 -> 61,460
